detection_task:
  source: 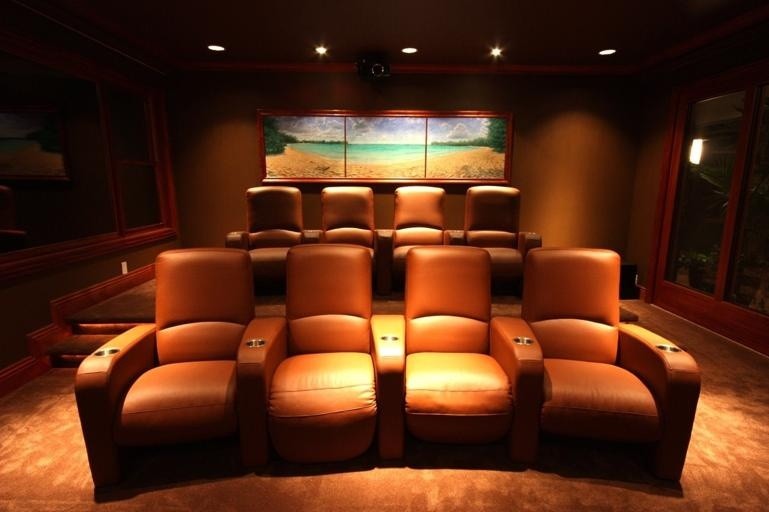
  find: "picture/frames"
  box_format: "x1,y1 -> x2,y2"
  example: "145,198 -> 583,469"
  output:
256,107 -> 515,190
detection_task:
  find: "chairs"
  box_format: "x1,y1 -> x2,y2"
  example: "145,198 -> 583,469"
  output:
405,245 -> 546,464
302,186 -> 375,298
374,185 -> 447,295
523,247 -> 702,488
268,243 -> 404,463
445,186 -> 542,300
226,184 -> 303,296
73,246 -> 289,490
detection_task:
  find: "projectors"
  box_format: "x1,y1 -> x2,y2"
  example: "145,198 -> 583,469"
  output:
353,63 -> 391,80
